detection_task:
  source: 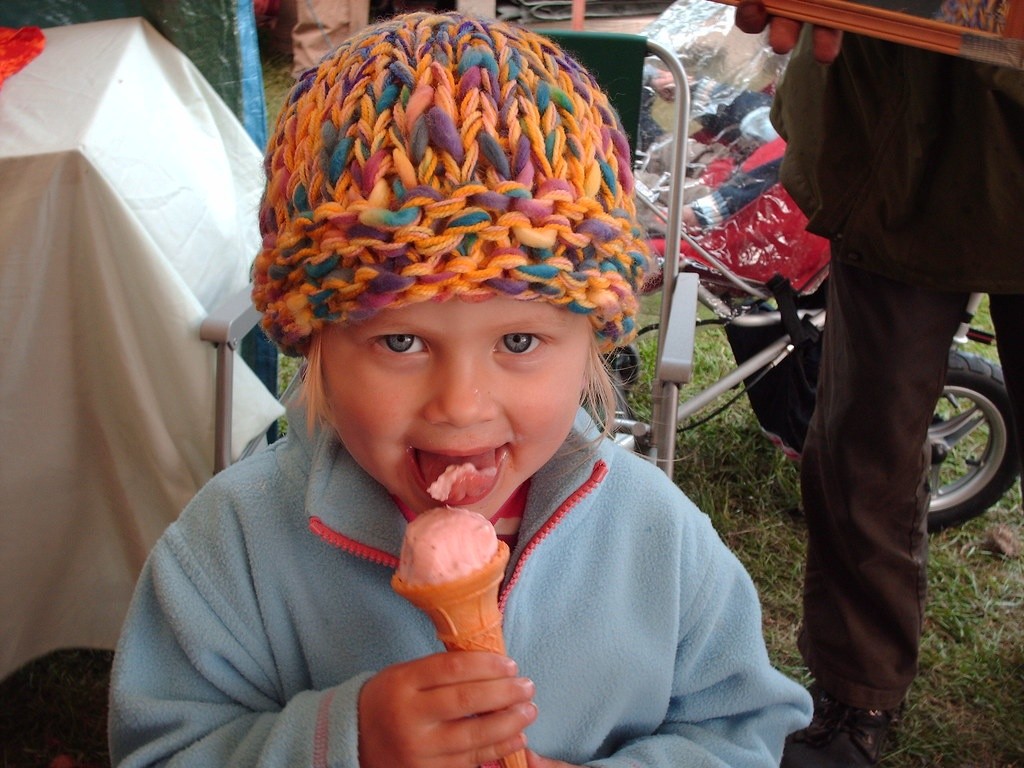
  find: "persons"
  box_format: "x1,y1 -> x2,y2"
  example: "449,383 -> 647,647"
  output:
635,43 -> 788,237
735,0 -> 1024,768
107,12 -> 813,768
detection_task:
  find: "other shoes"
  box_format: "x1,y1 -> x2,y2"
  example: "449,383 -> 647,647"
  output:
779,704 -> 897,768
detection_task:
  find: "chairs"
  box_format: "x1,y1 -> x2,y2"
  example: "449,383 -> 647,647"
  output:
198,27 -> 709,484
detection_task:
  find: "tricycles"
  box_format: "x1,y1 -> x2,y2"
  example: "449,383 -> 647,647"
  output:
613,161 -> 1020,533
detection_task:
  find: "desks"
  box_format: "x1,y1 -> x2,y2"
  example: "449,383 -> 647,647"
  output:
0,15 -> 297,682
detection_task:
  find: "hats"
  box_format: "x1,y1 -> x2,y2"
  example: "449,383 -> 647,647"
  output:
249,10 -> 658,358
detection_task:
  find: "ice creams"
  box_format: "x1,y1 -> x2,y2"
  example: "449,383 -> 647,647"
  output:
425,462 -> 476,502
392,505 -> 530,768
